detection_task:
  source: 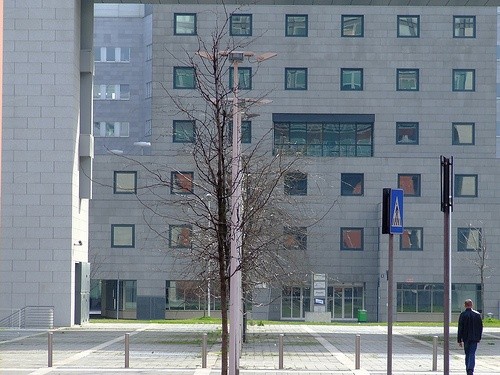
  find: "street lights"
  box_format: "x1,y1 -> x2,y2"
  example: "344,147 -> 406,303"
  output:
195,50 -> 276,373
205,193 -> 214,319
208,95 -> 273,358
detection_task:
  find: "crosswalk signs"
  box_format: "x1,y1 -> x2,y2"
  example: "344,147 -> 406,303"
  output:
382,187 -> 404,236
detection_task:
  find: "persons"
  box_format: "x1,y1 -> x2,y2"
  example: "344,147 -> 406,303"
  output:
457,299 -> 483,375
